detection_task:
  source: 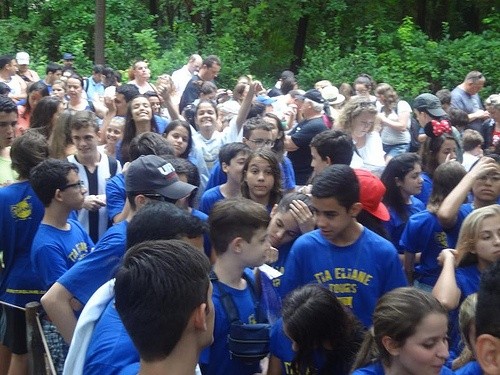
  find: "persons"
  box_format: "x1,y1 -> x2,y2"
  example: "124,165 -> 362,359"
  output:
0,51 -> 500,375
280,163 -> 408,330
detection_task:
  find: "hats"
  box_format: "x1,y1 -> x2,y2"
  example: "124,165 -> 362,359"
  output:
255,96 -> 277,106
125,154 -> 199,199
351,168 -> 391,221
413,92 -> 448,117
16,52 -> 30,65
63,53 -> 74,60
295,89 -> 325,103
318,86 -> 345,105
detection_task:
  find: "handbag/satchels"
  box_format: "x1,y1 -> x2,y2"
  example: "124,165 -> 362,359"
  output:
227,322 -> 271,360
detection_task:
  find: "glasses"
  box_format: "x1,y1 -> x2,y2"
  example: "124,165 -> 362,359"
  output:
355,87 -> 369,95
469,74 -> 484,80
61,180 -> 86,194
133,190 -> 177,205
247,138 -> 276,148
